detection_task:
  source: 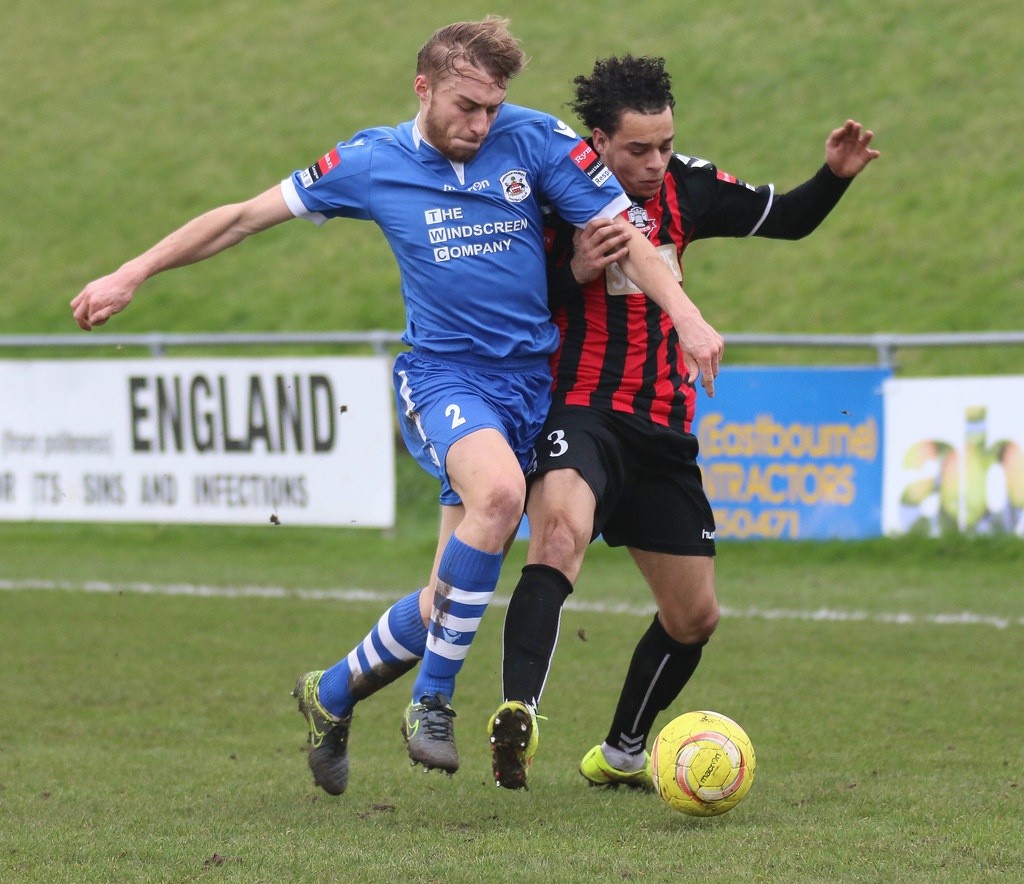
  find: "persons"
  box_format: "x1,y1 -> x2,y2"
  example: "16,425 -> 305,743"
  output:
488,51 -> 882,790
67,9 -> 726,795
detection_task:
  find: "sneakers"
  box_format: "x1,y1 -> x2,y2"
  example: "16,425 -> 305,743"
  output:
400,692 -> 460,779
486,700 -> 549,790
578,744 -> 655,791
290,669 -> 352,795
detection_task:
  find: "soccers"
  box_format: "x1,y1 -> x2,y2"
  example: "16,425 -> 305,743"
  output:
649,709 -> 757,818
